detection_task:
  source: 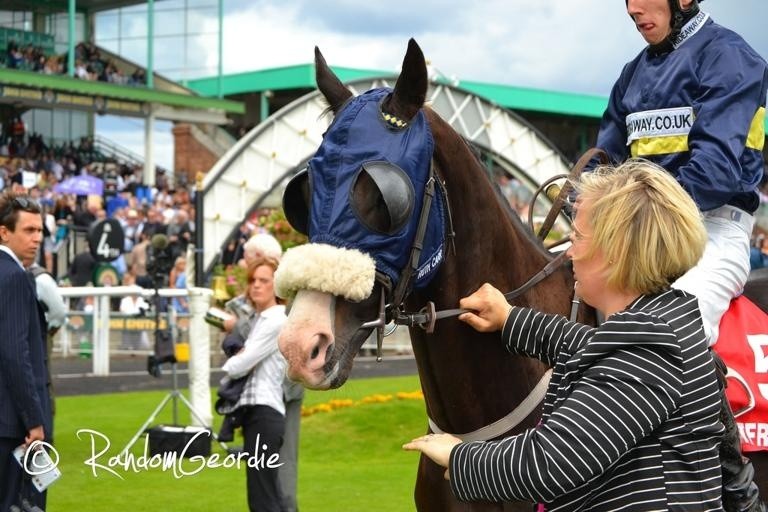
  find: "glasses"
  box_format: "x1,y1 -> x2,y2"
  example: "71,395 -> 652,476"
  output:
5,196 -> 41,216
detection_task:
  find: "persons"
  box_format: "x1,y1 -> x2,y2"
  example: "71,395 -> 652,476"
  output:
56,137 -> 219,356
221,232 -> 305,511
567,0 -> 768,350
0,38 -> 147,88
0,192 -> 55,512
401,156 -> 729,512
0,120 -> 55,204
219,257 -> 289,511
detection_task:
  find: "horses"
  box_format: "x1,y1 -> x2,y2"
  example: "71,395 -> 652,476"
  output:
277,38 -> 768,512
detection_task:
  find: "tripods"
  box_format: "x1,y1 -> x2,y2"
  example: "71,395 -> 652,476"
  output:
118,303 -> 228,462
